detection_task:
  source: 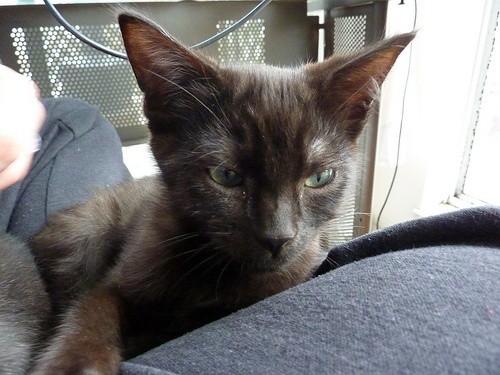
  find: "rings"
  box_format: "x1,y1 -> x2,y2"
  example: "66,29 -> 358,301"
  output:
28,133 -> 41,152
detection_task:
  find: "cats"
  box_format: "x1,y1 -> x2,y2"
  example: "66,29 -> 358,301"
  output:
0,3 -> 419,375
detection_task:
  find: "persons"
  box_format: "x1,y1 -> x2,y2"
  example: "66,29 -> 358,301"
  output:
0,62 -> 500,375
